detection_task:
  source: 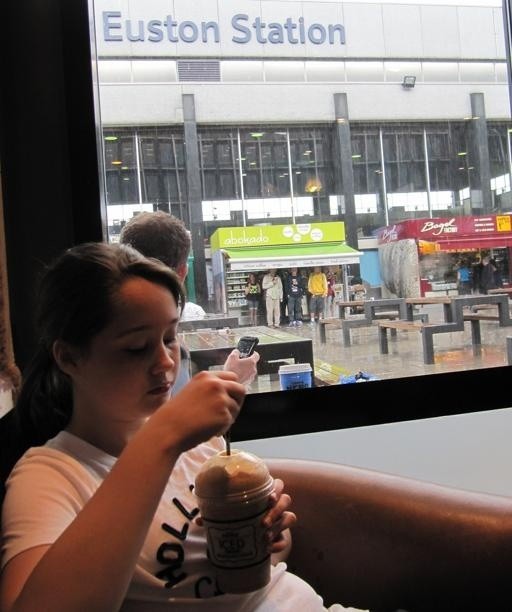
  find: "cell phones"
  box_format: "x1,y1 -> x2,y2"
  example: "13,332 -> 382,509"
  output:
236,336 -> 259,359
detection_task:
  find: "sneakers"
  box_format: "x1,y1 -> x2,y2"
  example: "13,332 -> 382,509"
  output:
250,318 -> 324,330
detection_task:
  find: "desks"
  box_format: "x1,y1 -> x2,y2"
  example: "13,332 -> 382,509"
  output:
227,404 -> 512,498
176,325 -> 315,389
175,313 -> 239,333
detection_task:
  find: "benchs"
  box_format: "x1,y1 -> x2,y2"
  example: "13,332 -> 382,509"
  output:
267,356 -> 369,385
231,456 -> 512,612
318,288 -> 512,365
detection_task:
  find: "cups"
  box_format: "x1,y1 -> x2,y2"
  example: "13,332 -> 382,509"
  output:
194,448 -> 275,595
277,363 -> 312,391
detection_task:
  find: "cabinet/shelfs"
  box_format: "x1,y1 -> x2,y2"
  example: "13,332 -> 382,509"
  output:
227,272 -> 252,327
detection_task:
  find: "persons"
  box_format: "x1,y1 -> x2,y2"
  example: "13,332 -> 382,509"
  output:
259,265 -> 363,330
244,272 -> 262,327
1,237 -> 374,610
451,255 -> 504,296
116,208 -> 195,297
